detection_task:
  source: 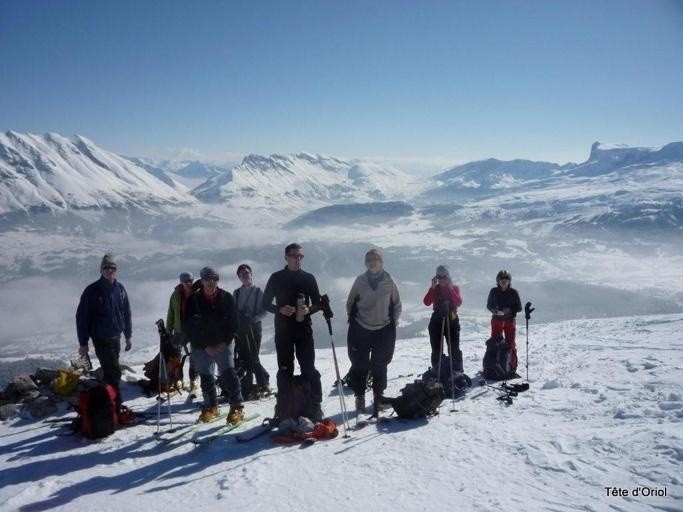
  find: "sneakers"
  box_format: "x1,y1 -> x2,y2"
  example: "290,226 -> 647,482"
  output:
200,404 -> 218,422
176,379 -> 197,390
227,406 -> 244,423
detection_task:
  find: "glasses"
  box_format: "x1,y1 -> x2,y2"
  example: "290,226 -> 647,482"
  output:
203,273 -> 219,280
436,274 -> 447,279
238,272 -> 250,277
184,279 -> 193,283
102,265 -> 116,272
287,254 -> 304,258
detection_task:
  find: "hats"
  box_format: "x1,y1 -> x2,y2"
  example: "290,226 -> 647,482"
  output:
180,272 -> 194,281
436,265 -> 448,275
101,254 -> 117,272
200,267 -> 219,280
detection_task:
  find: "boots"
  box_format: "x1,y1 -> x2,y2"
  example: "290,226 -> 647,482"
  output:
356,393 -> 365,410
374,392 -> 385,410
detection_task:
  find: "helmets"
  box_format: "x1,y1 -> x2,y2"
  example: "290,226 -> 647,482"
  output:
496,269 -> 511,281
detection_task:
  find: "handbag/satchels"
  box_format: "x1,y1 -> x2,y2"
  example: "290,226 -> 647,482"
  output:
347,360 -> 375,388
141,350 -> 183,384
272,370 -> 323,422
221,358 -> 253,400
77,384 -> 119,438
393,356 -> 472,420
483,337 -> 512,380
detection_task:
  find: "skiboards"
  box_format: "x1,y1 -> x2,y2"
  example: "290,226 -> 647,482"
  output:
156,385 -> 198,403
356,397 -> 381,424
44,408 -> 199,424
157,414 -> 258,444
236,418 -> 277,441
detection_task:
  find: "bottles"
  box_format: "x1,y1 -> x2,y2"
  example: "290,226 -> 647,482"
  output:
82,352 -> 93,370
295,291 -> 306,323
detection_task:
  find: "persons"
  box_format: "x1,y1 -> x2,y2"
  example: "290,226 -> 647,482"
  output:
346,249 -> 402,425
261,244 -> 321,433
488,269 -> 522,378
185,266 -> 243,425
422,265 -> 461,372
227,263 -> 271,398
165,271 -> 198,390
74,252 -> 132,426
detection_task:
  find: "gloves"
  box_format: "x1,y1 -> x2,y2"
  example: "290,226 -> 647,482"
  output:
511,383 -> 529,392
525,302 -> 535,319
321,294 -> 333,318
